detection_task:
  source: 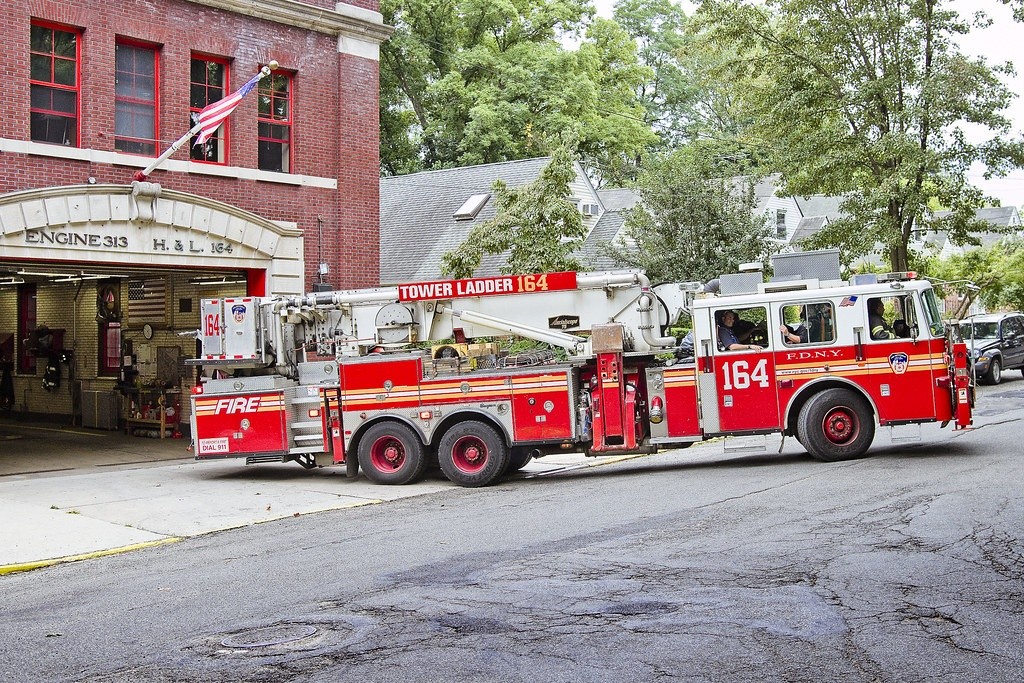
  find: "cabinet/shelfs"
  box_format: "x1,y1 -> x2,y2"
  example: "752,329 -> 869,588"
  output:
124,387 -> 182,439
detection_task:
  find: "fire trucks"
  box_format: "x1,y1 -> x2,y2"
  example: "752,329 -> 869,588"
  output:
187,249 -> 982,490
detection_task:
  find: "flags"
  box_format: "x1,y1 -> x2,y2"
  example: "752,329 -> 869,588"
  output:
192,75 -> 261,148
128,280 -> 165,323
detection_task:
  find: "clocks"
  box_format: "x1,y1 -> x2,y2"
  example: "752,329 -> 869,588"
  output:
143,323 -> 153,340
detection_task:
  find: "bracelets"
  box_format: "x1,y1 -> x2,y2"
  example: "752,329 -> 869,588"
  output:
748,346 -> 750,349
787,333 -> 790,337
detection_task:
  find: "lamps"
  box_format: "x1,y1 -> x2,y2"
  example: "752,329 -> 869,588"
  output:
187,278 -> 247,285
317,262 -> 330,281
87,177 -> 97,185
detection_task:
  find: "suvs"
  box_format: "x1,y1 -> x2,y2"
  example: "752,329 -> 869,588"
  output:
947,311 -> 1024,386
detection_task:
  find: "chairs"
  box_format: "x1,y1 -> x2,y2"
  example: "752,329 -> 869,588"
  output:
817,312 -> 827,342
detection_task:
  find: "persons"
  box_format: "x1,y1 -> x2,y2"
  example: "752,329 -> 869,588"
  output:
719,310 -> 763,353
871,300 -> 901,338
780,304 -> 820,343
